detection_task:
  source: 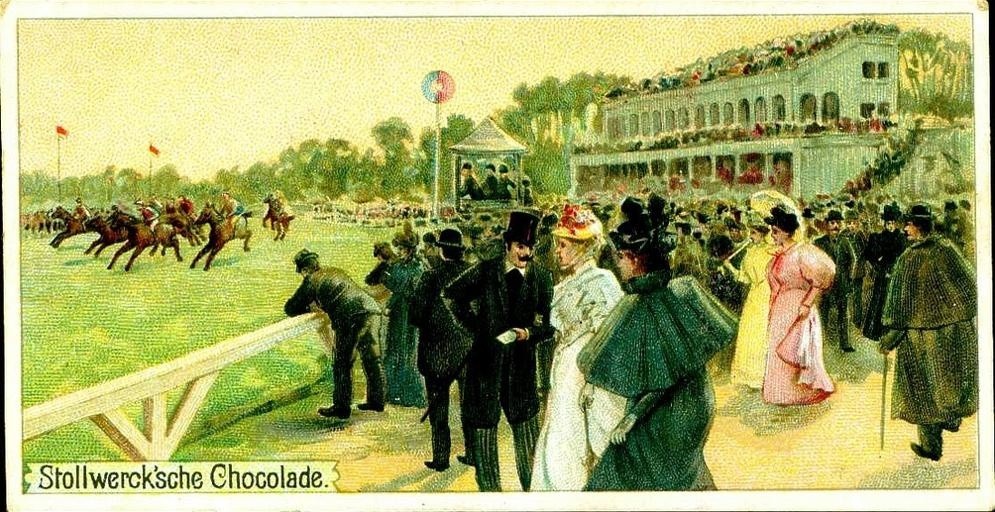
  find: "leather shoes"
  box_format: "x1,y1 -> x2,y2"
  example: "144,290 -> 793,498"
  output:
911,443 -> 939,461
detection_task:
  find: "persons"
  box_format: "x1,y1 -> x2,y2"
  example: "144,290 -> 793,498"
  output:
18,19 -> 979,490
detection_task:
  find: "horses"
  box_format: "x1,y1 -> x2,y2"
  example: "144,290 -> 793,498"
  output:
189,201 -> 253,271
48,200 -> 206,273
261,192 -> 294,242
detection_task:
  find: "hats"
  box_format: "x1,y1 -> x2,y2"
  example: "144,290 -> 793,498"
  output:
435,229 -> 466,251
904,204 -> 935,221
394,231 -> 419,248
551,204 -> 604,241
825,211 -> 844,221
503,211 -> 540,248
880,205 -> 902,221
293,248 -> 318,272
